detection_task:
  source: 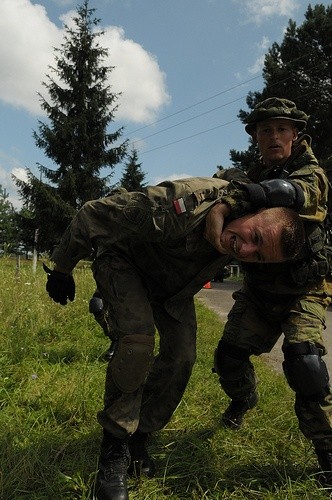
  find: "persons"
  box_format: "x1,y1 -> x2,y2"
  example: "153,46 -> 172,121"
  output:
206,97 -> 332,483
42,168 -> 305,500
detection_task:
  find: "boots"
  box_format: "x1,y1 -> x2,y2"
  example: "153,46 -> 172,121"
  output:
127,430 -> 155,479
96,428 -> 129,500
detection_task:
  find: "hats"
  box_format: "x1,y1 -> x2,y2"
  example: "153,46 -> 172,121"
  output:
245,97 -> 308,136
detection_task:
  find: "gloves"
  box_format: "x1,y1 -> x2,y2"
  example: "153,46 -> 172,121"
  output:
46,269 -> 75,305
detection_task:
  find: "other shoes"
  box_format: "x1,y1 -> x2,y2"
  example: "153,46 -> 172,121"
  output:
221,390 -> 259,430
315,450 -> 332,485
